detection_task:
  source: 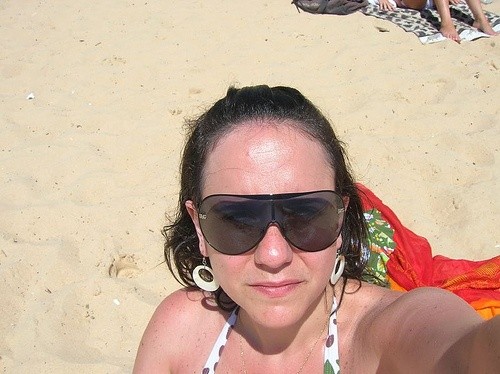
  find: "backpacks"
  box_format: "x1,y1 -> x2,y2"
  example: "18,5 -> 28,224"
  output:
291,0 -> 369,15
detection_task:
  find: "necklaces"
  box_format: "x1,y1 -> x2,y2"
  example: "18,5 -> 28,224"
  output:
237,306 -> 329,374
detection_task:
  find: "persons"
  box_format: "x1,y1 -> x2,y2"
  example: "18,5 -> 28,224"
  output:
378,0 -> 500,42
131,84 -> 500,374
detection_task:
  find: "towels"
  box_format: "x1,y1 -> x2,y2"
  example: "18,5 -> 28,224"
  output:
346,182 -> 500,320
358,0 -> 500,45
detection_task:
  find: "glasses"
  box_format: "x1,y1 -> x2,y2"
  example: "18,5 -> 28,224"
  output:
198,190 -> 345,255
